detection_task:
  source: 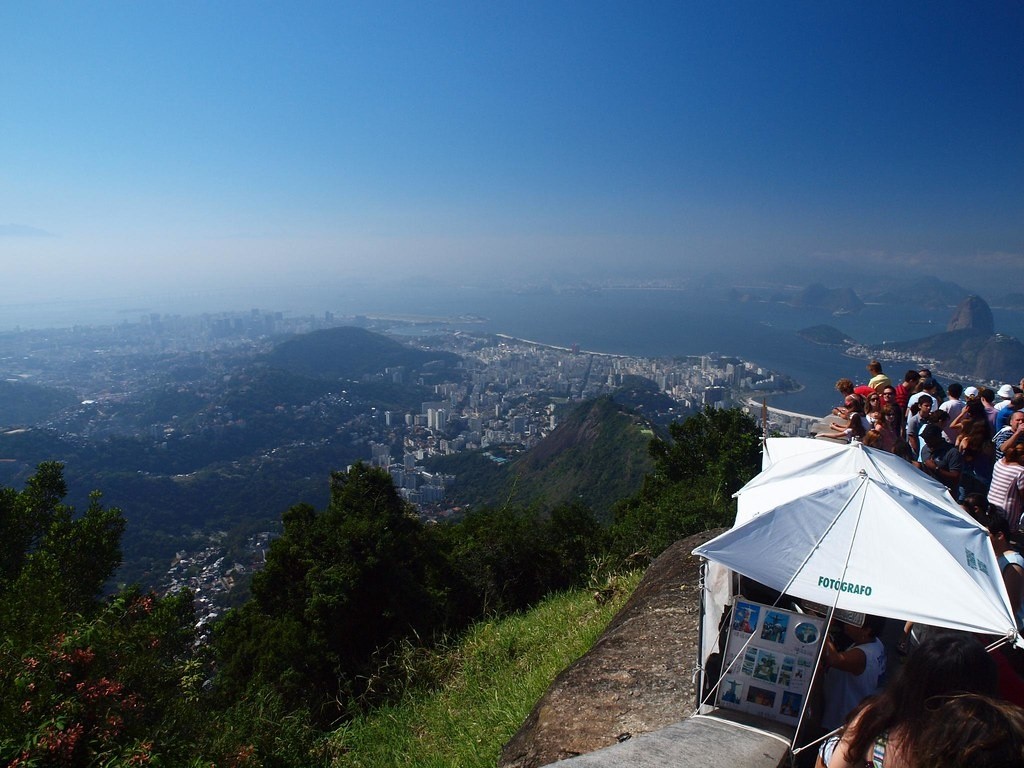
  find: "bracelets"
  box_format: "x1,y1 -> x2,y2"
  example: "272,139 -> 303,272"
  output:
837,411 -> 842,416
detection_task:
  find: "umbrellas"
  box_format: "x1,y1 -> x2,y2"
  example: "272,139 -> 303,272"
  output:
731,438 -> 990,534
691,470 -> 1024,650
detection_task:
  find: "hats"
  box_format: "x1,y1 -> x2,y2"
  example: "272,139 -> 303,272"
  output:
997,384 -> 1014,398
964,386 -> 978,398
919,424 -> 942,438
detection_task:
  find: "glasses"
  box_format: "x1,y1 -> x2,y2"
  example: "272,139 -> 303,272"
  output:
870,398 -> 878,402
844,404 -> 853,410
883,392 -> 893,395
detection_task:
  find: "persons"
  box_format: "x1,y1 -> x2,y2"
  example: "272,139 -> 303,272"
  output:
733,617 -> 750,633
761,621 -> 786,644
756,693 -> 770,706
814,360 -> 1023,633
781,702 -> 791,715
814,615 -> 1024,768
797,626 -> 816,644
755,658 -> 773,681
